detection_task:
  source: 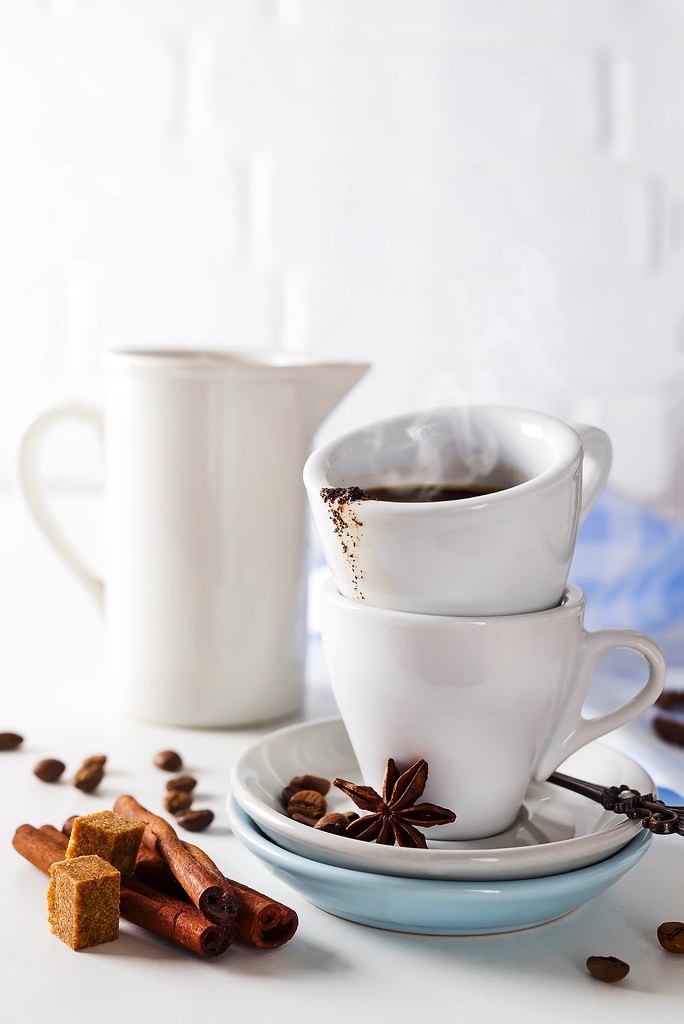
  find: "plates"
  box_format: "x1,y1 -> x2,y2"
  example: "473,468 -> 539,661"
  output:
228,716 -> 660,882
224,794 -> 654,939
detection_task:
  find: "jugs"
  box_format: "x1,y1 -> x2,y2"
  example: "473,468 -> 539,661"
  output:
17,346 -> 374,732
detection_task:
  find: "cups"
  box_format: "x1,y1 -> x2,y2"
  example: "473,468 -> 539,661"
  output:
317,574 -> 667,843
301,402 -> 614,618
